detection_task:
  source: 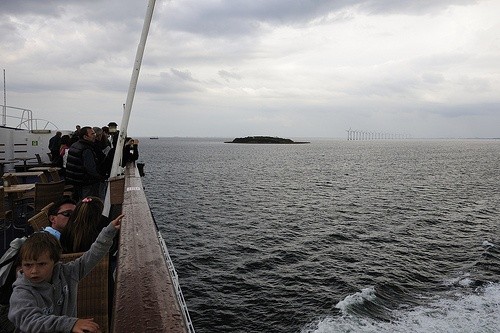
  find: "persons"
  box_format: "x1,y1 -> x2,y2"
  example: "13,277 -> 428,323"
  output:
48,124 -> 139,201
41,197 -> 77,253
60,195 -> 118,332
7,212 -> 126,333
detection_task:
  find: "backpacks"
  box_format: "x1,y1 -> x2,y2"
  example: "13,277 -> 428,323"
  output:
0,231 -> 48,305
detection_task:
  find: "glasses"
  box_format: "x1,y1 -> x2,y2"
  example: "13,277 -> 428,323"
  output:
54,209 -> 75,217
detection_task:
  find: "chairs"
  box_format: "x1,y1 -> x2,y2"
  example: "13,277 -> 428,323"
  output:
35,153 -> 53,168
29,203 -> 110,333
0,172 -> 73,250
38,167 -> 79,202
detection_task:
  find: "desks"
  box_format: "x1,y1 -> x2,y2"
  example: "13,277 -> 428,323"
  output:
0,156 -> 37,172
0,167 -> 64,216
